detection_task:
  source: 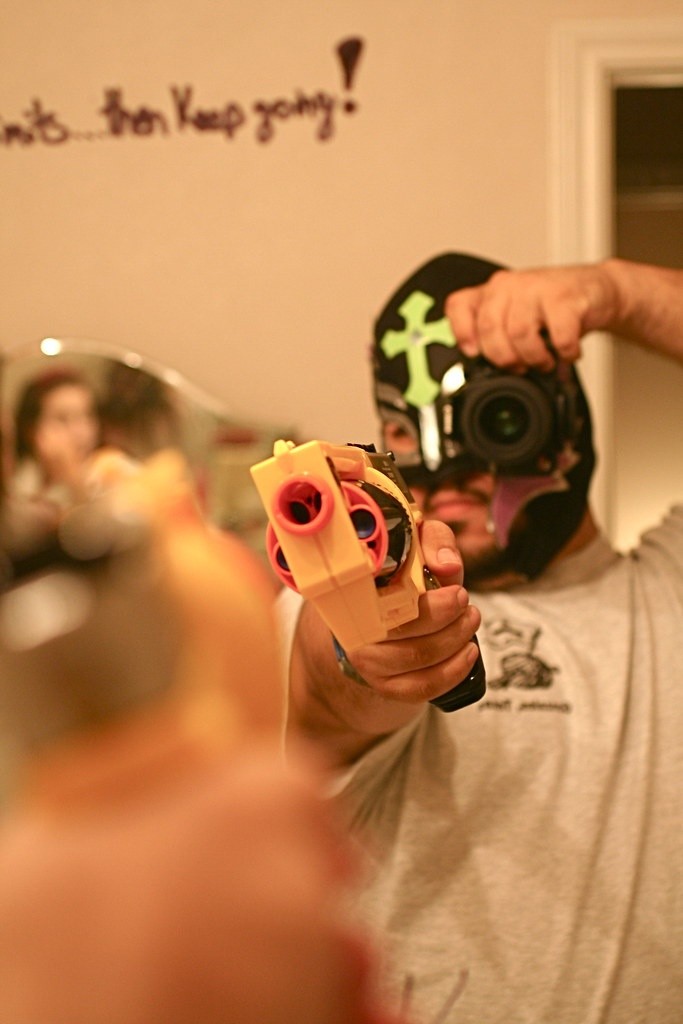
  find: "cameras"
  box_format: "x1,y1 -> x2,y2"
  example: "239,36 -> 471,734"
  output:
452,326 -> 566,476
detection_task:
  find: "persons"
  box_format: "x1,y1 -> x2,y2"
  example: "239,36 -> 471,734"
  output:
0,451 -> 370,1024
273,250 -> 683,1024
9,371 -> 140,508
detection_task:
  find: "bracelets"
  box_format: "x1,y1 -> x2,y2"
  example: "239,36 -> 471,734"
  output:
332,632 -> 372,687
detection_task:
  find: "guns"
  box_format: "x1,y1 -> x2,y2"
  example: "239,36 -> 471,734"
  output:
243,438 -> 492,720
